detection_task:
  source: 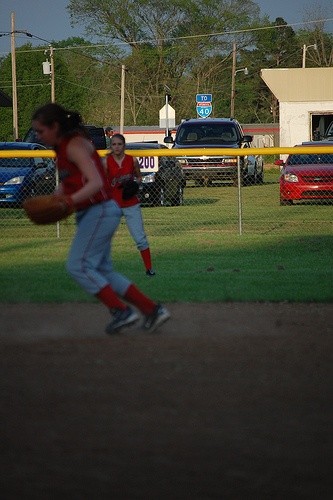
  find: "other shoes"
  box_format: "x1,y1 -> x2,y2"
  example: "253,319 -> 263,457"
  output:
147,270 -> 155,276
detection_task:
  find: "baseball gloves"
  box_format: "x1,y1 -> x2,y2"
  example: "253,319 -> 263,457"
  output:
118,180 -> 140,202
21,196 -> 72,226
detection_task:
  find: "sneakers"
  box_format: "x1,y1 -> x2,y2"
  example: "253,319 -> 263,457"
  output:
104,308 -> 139,332
143,308 -> 170,331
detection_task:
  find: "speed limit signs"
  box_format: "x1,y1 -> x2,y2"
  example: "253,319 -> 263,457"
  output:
196,102 -> 212,118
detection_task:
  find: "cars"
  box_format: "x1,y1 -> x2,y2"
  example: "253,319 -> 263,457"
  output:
123,142 -> 187,208
247,147 -> 264,185
273,141 -> 333,207
0,141 -> 57,209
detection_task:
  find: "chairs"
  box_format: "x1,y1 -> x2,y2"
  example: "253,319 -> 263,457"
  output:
184,132 -> 199,141
221,126 -> 234,138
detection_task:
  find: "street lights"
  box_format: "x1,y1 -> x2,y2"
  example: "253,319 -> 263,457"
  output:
231,42 -> 249,118
302,43 -> 318,68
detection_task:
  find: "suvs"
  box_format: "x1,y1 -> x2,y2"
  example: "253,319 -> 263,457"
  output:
14,125 -> 107,159
164,117 -> 253,187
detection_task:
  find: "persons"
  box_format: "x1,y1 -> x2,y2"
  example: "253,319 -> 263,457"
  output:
104,127 -> 113,150
101,133 -> 156,276
20,104 -> 171,333
